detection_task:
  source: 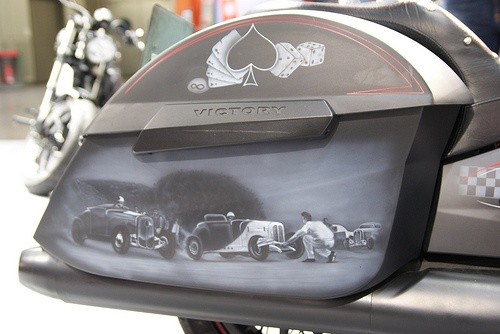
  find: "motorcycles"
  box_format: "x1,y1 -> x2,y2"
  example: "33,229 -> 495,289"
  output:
8,0 -> 148,195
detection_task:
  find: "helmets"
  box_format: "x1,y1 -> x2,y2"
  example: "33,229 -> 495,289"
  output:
225,210 -> 235,217
117,196 -> 125,202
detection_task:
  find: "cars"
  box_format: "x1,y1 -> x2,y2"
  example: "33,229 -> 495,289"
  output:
71,202 -> 176,260
184,212 -> 306,261
330,221 -> 383,249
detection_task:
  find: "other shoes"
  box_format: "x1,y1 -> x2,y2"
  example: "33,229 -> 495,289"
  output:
327,251 -> 337,263
302,258 -> 315,262
